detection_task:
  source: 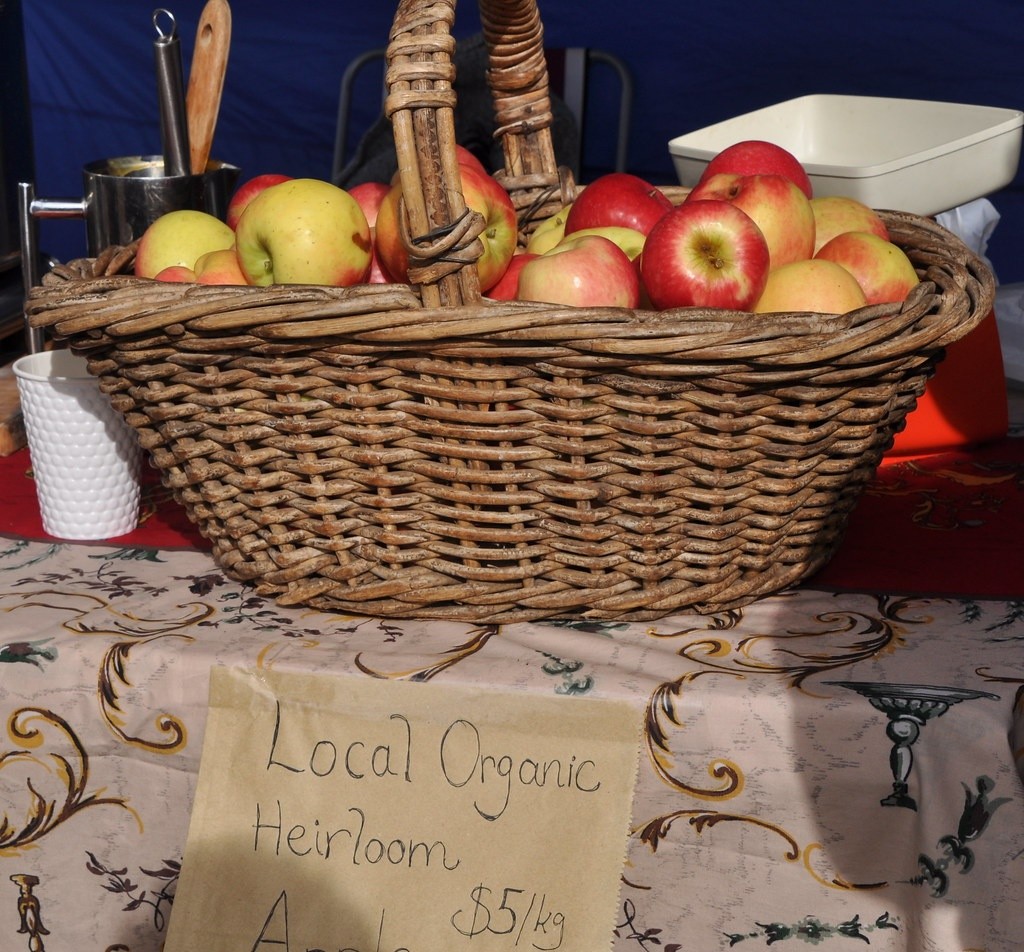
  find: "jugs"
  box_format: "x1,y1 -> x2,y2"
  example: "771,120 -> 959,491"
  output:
17,152 -> 240,358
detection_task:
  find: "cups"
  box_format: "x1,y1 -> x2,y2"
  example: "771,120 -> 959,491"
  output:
11,349 -> 141,540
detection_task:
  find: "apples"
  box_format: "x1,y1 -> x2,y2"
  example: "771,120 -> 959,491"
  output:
132,144 -> 920,315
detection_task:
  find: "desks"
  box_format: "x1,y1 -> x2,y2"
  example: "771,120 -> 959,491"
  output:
0,287 -> 1024,952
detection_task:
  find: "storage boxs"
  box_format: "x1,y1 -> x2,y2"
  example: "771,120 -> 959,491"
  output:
668,93 -> 1024,217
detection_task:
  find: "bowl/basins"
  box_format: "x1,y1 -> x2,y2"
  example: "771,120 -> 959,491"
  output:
667,88 -> 1023,215
